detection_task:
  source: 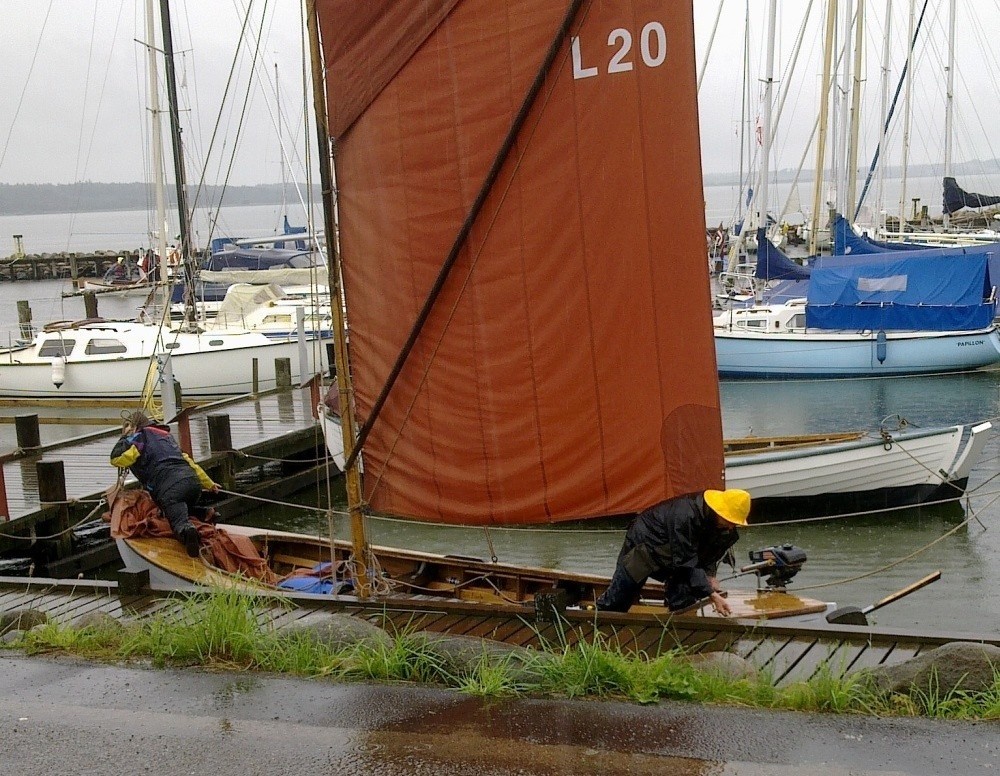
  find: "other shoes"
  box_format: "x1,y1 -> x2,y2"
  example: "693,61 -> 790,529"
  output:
183,526 -> 201,558
204,507 -> 219,526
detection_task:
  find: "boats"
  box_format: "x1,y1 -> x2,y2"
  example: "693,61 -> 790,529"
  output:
314,402 -> 994,528
84,251 -> 146,291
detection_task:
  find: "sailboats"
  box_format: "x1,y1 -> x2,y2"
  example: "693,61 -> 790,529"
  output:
0,0 -> 347,400
101,0 -> 837,626
692,0 -> 1000,379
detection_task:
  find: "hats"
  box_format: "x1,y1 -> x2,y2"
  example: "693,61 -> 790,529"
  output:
703,489 -> 752,526
123,411 -> 156,430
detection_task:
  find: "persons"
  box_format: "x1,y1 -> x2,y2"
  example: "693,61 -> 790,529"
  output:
168,244 -> 180,276
103,257 -> 132,285
142,248 -> 162,281
593,489 -> 752,616
110,411 -> 222,558
